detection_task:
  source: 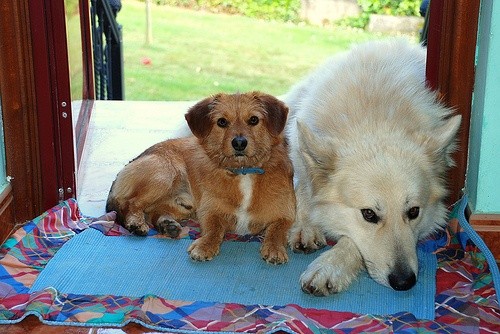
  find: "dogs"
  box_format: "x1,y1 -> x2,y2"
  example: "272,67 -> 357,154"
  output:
278,35 -> 464,297
106,91 -> 297,266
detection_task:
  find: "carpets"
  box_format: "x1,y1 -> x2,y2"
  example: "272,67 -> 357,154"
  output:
1,198 -> 500,334
